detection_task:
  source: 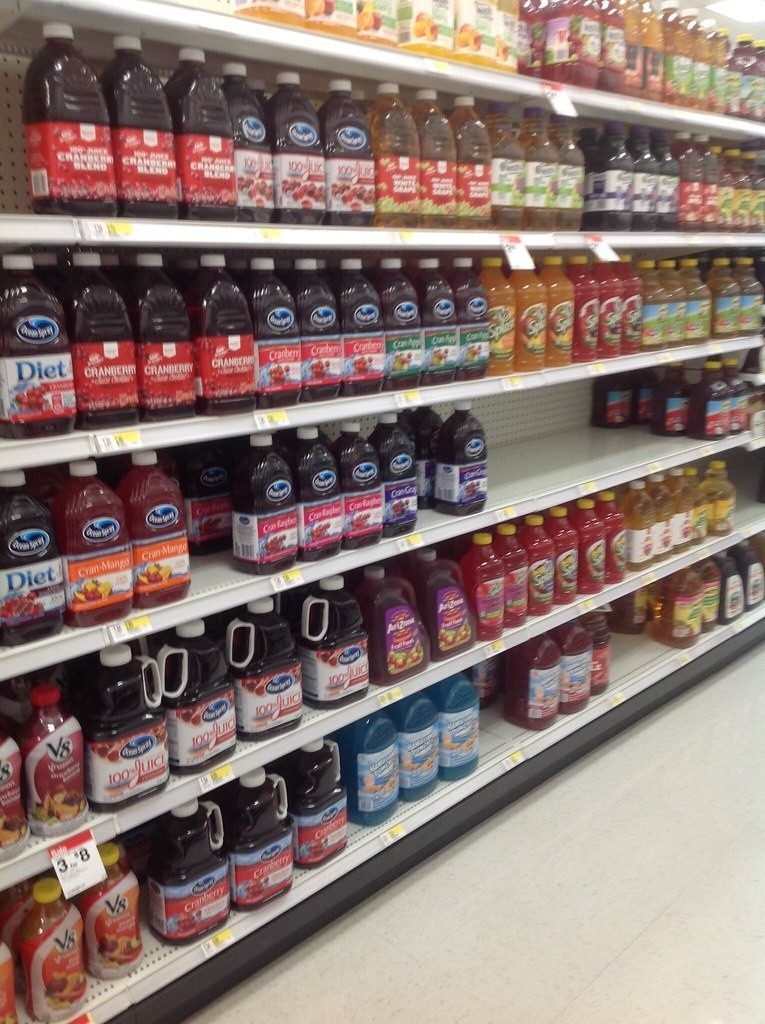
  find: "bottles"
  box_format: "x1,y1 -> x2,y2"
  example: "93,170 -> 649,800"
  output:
0,400 -> 765,1024
234,0 -> 765,124
25,18 -> 765,231
0,243 -> 765,440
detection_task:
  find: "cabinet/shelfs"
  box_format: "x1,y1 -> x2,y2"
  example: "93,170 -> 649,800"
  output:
0,0 -> 765,1024
